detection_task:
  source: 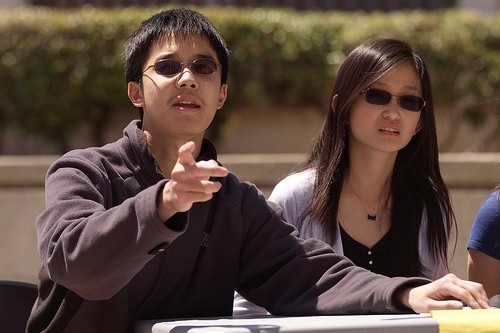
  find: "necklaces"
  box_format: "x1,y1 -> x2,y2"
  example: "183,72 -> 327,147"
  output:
343,175 -> 392,220
151,152 -> 167,179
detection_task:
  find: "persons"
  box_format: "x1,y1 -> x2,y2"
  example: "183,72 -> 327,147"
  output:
267,37 -> 459,281
466,184 -> 500,307
25,10 -> 491,333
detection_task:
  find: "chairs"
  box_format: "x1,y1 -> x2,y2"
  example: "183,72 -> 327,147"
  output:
0,281 -> 37,333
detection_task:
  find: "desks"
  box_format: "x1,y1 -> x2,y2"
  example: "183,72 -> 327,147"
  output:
134,304 -> 500,333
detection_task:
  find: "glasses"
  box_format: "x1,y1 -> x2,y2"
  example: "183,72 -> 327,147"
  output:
357,88 -> 426,111
136,58 -> 222,81
